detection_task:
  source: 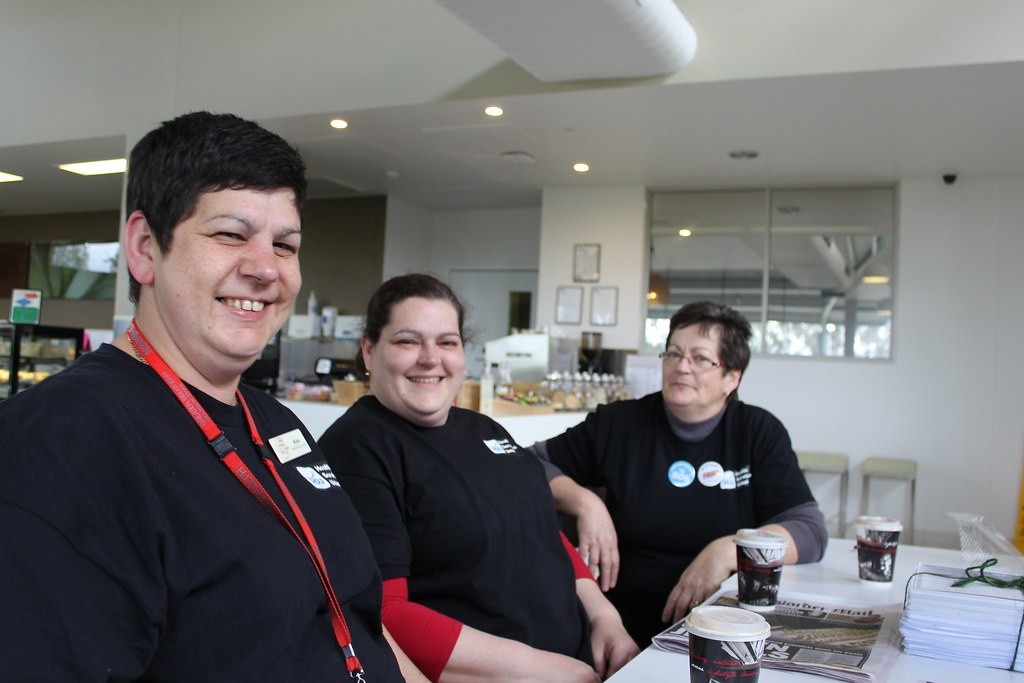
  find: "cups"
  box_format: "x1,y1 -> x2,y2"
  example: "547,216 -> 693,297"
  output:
322,307 -> 337,338
732,528 -> 787,611
684,606 -> 772,683
856,516 -> 903,585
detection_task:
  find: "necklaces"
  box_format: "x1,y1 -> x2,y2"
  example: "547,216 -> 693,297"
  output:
128,336 -> 147,365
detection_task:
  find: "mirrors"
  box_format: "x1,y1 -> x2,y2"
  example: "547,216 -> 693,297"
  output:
643,186 -> 895,358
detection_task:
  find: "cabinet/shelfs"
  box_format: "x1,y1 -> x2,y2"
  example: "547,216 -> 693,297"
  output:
0,321 -> 85,402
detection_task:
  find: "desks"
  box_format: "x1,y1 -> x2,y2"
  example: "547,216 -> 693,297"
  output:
602,535 -> 1024,683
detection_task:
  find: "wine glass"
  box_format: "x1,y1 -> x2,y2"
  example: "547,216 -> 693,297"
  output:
581,331 -> 602,375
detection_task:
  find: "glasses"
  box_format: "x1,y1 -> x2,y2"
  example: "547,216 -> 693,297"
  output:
659,351 -> 724,375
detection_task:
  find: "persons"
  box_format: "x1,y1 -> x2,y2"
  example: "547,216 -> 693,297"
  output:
0,110 -> 431,683
316,274 -> 640,683
523,301 -> 828,652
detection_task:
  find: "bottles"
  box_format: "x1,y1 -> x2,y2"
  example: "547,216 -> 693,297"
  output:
489,363 -> 498,389
495,361 -> 514,396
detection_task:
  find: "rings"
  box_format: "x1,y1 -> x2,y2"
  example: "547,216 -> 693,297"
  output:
691,601 -> 701,604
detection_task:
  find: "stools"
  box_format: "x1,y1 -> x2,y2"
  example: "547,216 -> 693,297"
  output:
795,451 -> 848,539
860,456 -> 917,546
